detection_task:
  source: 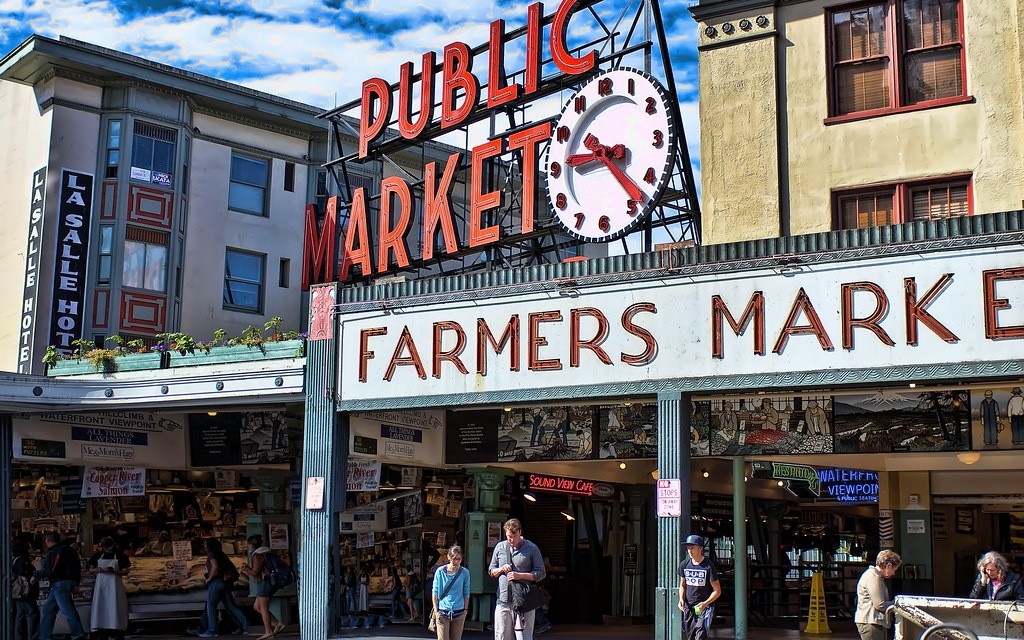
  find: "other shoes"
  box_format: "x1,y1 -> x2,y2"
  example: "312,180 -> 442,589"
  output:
199,631 -> 218,637
232,628 -> 248,635
273,623 -> 286,634
408,618 -> 415,623
256,633 -> 274,640
413,613 -> 419,619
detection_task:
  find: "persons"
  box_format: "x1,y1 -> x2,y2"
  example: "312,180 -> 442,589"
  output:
404,565 -> 419,622
31,532 -> 87,640
11,543 -> 39,640
677,535 -> 722,640
199,538 -> 247,637
488,518 -> 547,640
241,534 -> 287,640
87,536 -> 131,640
970,552 -> 1024,603
390,567 -> 406,618
146,522 -> 213,556
432,546 -> 471,640
854,550 -> 901,640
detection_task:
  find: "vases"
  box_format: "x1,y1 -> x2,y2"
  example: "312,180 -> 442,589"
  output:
47,352 -> 164,378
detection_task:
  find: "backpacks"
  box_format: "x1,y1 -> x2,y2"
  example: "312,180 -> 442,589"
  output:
261,551 -> 294,586
217,552 -> 240,582
412,575 -> 424,594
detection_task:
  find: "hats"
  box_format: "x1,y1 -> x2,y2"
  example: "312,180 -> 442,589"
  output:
100,536 -> 115,547
408,571 -> 414,575
681,535 -> 704,547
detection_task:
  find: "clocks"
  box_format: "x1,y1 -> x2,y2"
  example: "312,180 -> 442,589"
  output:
545,66 -> 678,244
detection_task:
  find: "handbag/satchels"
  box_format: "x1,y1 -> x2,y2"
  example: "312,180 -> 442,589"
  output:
11,572 -> 29,598
428,608 -> 436,632
511,578 -> 549,612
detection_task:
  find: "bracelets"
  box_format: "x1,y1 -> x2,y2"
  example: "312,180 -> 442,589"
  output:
465,609 -> 468,610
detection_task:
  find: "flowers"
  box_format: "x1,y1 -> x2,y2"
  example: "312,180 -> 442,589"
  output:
42,316 -> 309,370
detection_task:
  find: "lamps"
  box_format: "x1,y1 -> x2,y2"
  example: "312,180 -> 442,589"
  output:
14,477 -> 23,492
956,453 -> 980,465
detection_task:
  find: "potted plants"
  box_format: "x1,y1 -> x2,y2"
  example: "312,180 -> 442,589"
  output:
163,339 -> 305,376
